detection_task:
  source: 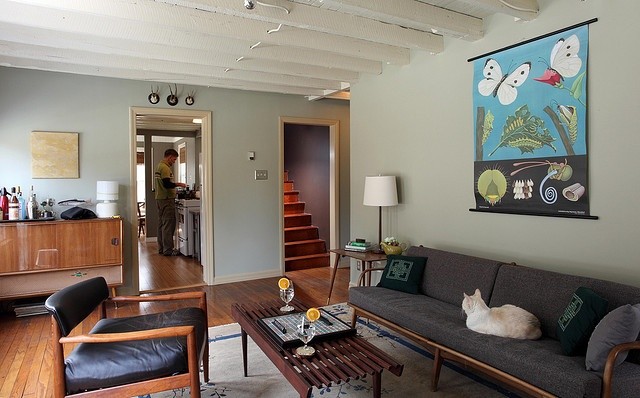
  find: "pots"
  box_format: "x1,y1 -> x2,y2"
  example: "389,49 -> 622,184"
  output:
176,190 -> 196,199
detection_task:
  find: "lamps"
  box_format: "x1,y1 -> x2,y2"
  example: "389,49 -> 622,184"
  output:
96,181 -> 119,219
363,176 -> 398,254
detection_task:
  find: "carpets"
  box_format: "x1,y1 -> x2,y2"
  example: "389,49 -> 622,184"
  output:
128,301 -> 507,397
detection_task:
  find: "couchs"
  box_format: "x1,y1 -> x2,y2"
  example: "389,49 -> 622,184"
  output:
347,245 -> 640,398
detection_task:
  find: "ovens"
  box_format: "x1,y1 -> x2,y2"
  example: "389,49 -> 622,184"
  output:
177,207 -> 189,257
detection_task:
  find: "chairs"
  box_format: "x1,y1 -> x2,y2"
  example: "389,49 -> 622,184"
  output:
137,202 -> 145,238
44,277 -> 209,398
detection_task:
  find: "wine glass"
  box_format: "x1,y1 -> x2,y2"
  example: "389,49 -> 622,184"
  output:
279,281 -> 295,313
296,313 -> 316,356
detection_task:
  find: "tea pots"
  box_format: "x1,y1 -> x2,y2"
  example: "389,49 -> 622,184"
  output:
380,241 -> 408,257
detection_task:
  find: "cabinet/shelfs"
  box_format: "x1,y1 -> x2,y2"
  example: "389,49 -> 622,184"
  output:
0,218 -> 124,309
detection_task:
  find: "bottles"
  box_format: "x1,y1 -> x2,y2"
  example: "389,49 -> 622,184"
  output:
33,194 -> 38,211
0,187 -> 9,220
27,185 -> 38,219
0,207 -> 4,221
16,186 -> 25,219
7,187 -> 19,220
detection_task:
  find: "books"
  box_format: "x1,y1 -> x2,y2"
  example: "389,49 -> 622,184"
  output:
344,241 -> 372,253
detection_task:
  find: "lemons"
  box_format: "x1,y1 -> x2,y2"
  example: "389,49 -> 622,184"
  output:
277,278 -> 289,290
307,308 -> 320,321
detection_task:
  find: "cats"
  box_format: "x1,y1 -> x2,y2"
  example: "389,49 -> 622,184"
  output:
461,288 -> 543,340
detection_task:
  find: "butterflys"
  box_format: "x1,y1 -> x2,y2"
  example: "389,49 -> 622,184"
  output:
538,34 -> 583,81
477,58 -> 532,107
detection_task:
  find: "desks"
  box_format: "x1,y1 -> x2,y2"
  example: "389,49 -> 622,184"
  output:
326,244 -> 402,305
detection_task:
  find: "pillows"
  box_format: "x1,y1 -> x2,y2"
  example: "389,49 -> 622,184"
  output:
586,303 -> 640,371
557,286 -> 609,356
376,254 -> 428,294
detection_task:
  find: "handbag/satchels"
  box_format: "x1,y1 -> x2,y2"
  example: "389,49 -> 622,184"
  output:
61,207 -> 97,219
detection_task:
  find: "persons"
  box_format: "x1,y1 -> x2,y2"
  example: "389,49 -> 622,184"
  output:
154,149 -> 186,256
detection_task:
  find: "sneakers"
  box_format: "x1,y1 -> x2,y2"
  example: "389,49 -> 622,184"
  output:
164,249 -> 179,256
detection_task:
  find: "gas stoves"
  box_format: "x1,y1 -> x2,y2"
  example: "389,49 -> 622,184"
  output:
175,198 -> 200,207
